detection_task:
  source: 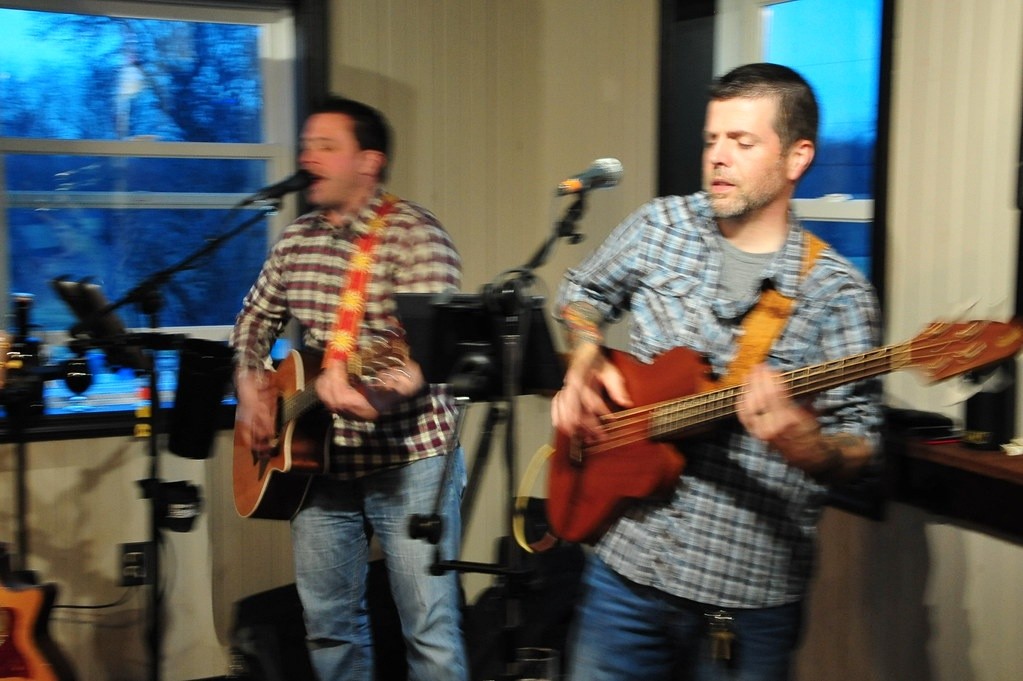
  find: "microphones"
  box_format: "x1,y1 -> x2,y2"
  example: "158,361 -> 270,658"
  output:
557,158 -> 622,197
241,169 -> 314,205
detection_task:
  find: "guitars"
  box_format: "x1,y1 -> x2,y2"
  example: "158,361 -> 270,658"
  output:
547,292 -> 1023,545
0,328 -> 75,681
232,325 -> 414,522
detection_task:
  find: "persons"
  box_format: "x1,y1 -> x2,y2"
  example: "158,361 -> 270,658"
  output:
230,96 -> 472,680
552,62 -> 884,680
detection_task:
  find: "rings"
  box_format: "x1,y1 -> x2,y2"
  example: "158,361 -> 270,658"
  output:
756,409 -> 767,415
